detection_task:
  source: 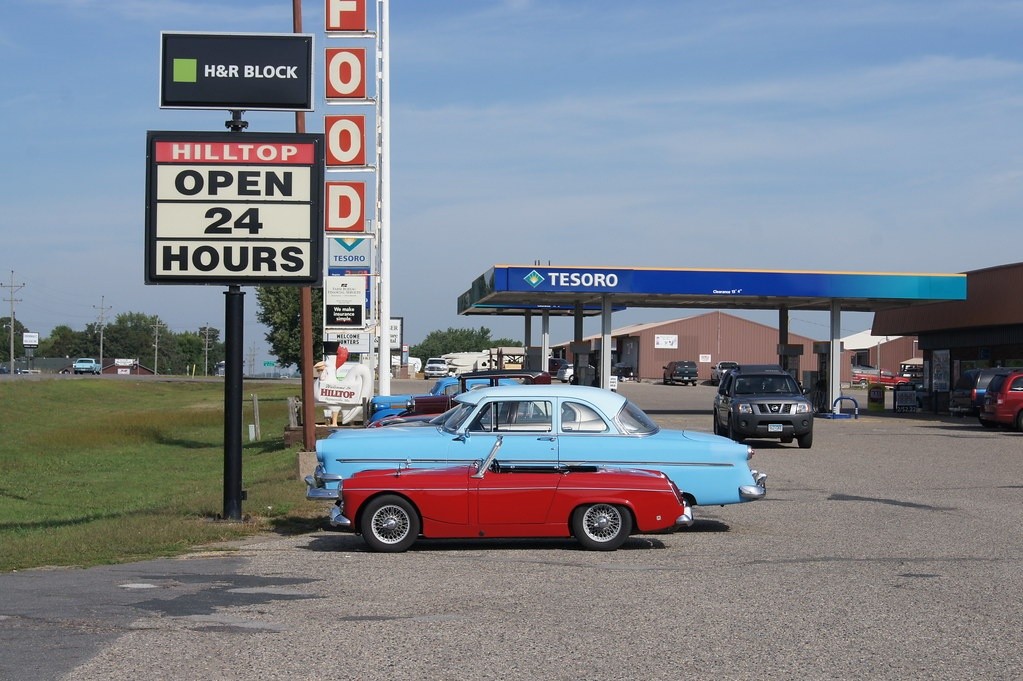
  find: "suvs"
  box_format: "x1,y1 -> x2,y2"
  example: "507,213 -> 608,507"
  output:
423,357 -> 450,380
661,360 -> 701,385
949,368 -> 1017,415
714,365 -> 815,450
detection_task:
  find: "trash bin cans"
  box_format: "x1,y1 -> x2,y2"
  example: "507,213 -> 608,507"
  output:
400,365 -> 408,380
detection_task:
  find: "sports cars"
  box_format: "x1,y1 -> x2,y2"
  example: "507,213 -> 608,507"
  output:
331,438 -> 696,552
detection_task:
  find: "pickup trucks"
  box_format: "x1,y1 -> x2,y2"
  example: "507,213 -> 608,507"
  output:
852,367 -> 912,385
711,361 -> 740,384
73,358 -> 102,375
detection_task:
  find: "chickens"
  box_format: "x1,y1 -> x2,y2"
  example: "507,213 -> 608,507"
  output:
313,340 -> 370,428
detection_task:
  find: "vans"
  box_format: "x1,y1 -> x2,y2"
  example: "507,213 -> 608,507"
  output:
214,362 -> 227,378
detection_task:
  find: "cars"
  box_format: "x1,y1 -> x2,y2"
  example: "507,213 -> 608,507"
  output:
548,358 -> 570,373
365,368 -> 637,433
308,382 -> 768,508
558,364 -> 597,384
980,371 -> 1023,432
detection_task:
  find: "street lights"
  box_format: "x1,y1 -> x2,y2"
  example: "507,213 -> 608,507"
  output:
95,330 -> 104,375
3,324 -> 16,375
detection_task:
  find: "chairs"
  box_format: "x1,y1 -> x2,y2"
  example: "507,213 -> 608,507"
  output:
737,382 -> 749,391
